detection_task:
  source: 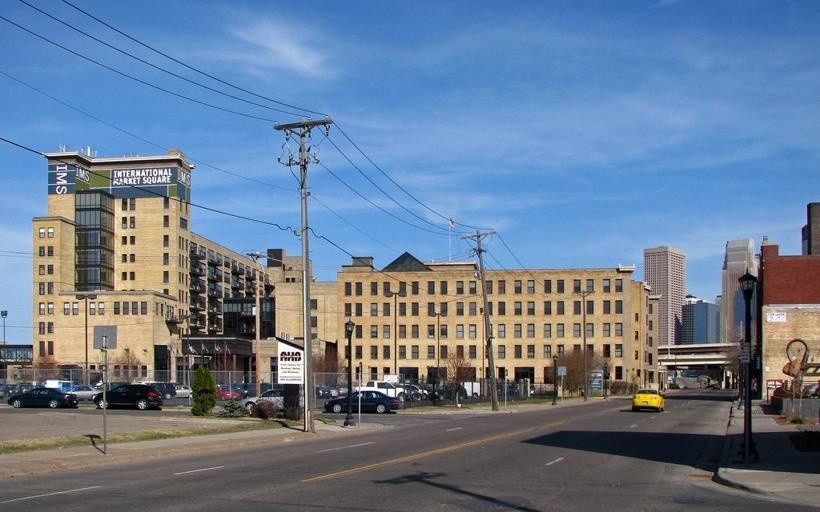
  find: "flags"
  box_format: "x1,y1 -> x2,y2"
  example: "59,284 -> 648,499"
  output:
166,342 -> 232,358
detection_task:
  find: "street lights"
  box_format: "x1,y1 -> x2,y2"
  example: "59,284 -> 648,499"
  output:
737,265 -> 757,463
1,309 -> 7,363
603,362 -> 609,400
551,353 -> 559,406
342,318 -> 356,429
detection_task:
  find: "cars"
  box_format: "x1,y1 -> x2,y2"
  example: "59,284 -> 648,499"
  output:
0,378 -> 195,410
312,379 -> 480,414
239,388 -> 305,417
214,383 -> 271,400
632,388 -> 665,413
489,382 -> 537,398
